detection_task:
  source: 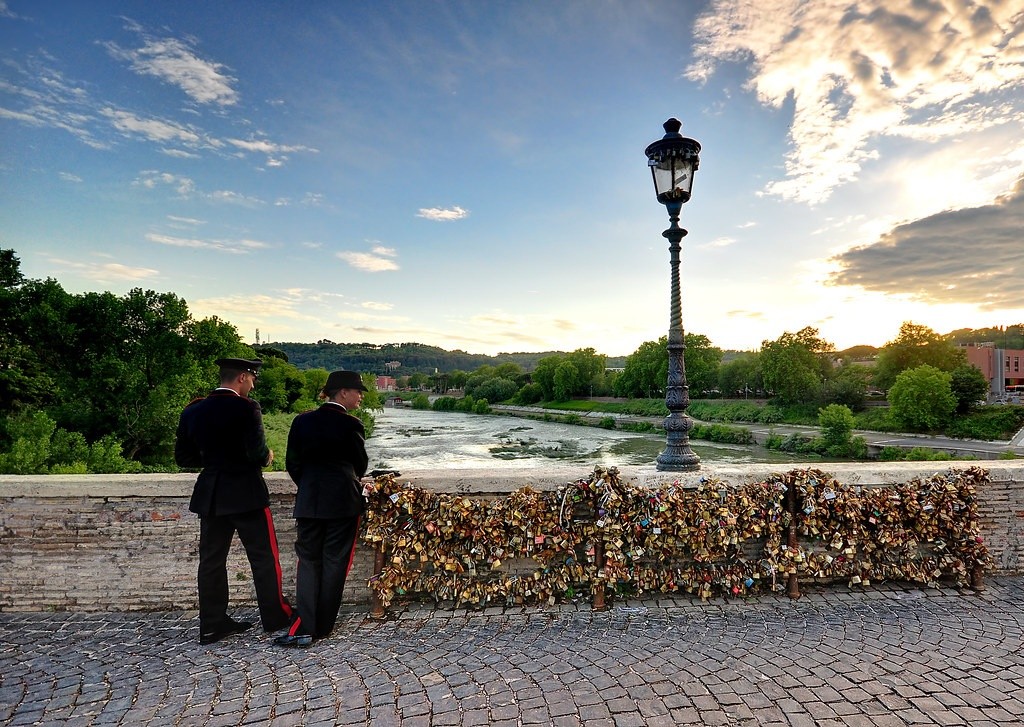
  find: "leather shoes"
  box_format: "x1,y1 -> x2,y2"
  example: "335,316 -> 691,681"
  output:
274,635 -> 312,646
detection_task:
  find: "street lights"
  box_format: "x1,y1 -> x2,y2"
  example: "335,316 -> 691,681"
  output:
645,117 -> 702,474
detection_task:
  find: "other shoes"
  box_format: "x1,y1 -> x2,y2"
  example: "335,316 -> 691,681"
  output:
199,611 -> 252,645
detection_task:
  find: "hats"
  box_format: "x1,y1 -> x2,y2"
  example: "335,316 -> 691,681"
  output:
214,358 -> 263,379
324,371 -> 368,392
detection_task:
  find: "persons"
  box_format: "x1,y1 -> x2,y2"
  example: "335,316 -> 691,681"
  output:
274,371 -> 369,645
175,358 -> 298,645
